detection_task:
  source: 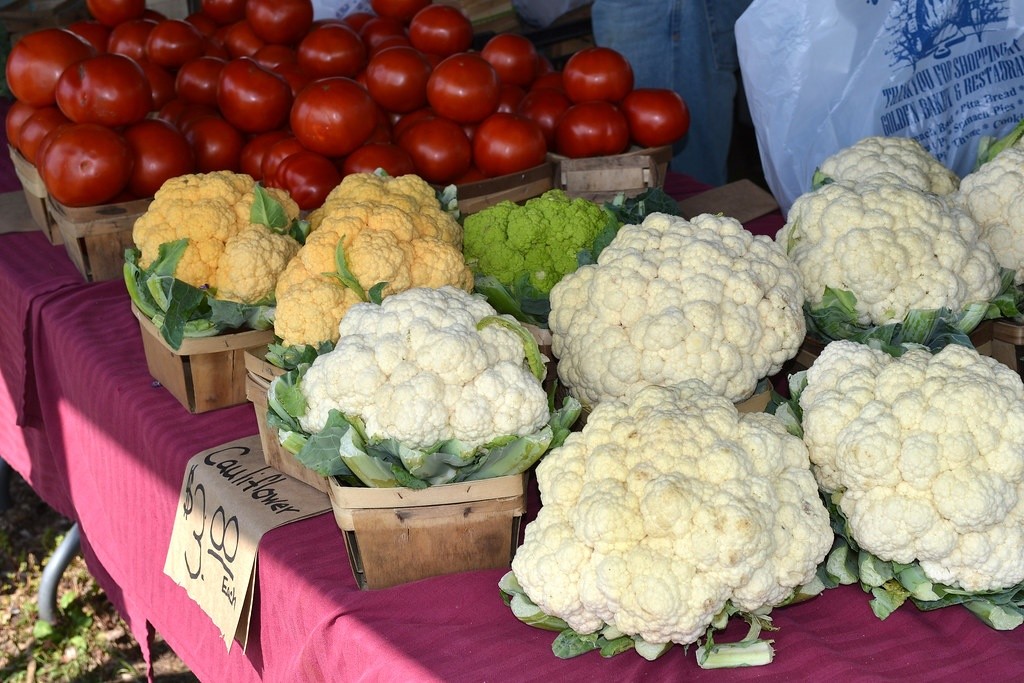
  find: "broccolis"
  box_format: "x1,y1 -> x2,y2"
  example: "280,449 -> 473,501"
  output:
124,136 -> 1024,662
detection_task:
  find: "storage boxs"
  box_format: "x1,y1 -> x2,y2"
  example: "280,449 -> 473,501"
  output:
6,145 -> 673,591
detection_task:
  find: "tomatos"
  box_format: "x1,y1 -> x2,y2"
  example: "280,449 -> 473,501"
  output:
5,0 -> 692,210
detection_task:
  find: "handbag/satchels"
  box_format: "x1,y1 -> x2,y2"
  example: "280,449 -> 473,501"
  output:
734,0 -> 1024,223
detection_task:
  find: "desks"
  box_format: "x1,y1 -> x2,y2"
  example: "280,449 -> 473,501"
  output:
0,156 -> 1024,683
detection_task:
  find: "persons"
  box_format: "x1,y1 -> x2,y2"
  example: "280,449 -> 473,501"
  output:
589,0 -> 753,187
